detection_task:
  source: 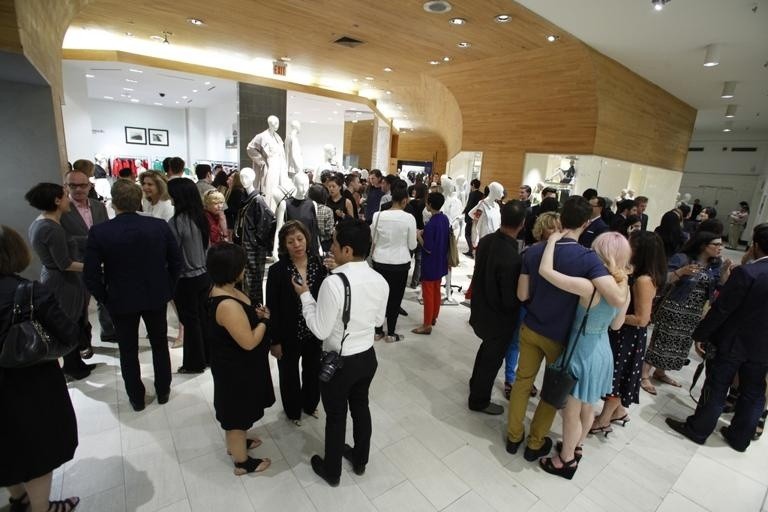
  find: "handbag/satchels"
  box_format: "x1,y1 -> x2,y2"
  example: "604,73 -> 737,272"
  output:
321,236 -> 334,252
366,256 -> 373,267
0,319 -> 79,367
703,350 -> 722,381
448,231 -> 461,267
539,367 -> 577,410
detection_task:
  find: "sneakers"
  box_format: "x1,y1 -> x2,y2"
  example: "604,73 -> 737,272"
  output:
524,437 -> 553,461
506,432 -> 525,454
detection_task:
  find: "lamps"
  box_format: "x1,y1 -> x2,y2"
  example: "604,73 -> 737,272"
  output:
703,44 -> 720,69
725,105 -> 737,118
721,80 -> 737,99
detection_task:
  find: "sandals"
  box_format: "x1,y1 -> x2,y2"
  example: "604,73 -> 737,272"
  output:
640,378 -> 656,394
9,492 -> 30,512
653,372 -> 676,386
227,438 -> 261,456
48,496 -> 79,512
504,382 -> 513,400
531,385 -> 537,397
234,456 -> 271,475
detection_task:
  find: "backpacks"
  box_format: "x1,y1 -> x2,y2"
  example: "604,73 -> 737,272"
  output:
260,209 -> 277,251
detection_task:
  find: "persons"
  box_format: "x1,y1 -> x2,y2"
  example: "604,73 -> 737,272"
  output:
246,115 -> 296,209
1,158 -> 767,511
313,145 -> 342,183
287,123 -> 302,189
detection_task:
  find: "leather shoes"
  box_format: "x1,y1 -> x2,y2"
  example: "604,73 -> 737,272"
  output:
665,417 -> 708,444
311,409 -> 320,419
311,455 -> 340,487
343,444 -> 366,475
80,347 -> 93,359
721,426 -> 745,452
178,367 -> 202,373
469,401 -> 504,415
172,338 -> 183,348
155,388 -> 169,404
374,332 -> 384,341
128,394 -> 145,412
292,419 -> 302,427
386,334 -> 404,342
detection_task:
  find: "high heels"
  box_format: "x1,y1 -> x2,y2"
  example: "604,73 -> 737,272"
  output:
412,326 -> 433,335
432,319 -> 436,325
610,414 -> 630,426
539,454 -> 577,480
80,364 -> 96,371
556,441 -> 583,463
589,424 -> 613,437
63,366 -> 90,382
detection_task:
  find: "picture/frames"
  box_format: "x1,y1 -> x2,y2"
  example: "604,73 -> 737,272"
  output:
148,128 -> 169,146
125,126 -> 147,145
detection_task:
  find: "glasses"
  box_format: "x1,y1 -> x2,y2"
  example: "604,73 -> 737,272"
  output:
207,188 -> 221,196
65,182 -> 91,189
704,240 -> 723,246
